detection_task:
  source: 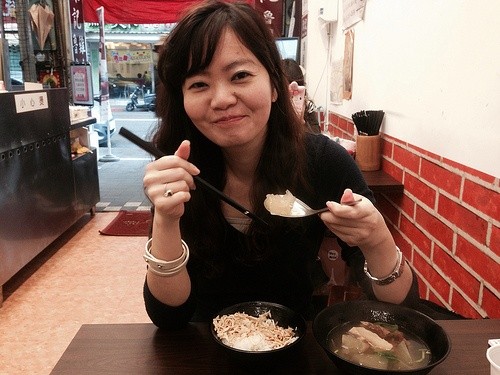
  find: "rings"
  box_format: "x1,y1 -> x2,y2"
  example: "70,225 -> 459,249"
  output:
164,182 -> 173,198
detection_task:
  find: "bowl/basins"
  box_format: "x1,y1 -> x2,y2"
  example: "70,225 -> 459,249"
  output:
486,344 -> 500,375
312,299 -> 451,375
211,300 -> 306,354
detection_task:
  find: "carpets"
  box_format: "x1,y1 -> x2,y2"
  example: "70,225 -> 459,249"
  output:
99,211 -> 153,237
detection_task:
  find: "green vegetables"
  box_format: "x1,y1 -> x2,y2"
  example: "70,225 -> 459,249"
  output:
376,322 -> 399,361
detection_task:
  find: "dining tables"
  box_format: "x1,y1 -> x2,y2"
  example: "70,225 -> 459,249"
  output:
49,319 -> 500,375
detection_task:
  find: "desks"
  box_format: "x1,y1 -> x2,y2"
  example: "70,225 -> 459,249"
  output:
361,170 -> 404,192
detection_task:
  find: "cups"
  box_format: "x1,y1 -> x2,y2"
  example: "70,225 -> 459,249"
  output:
356,134 -> 381,171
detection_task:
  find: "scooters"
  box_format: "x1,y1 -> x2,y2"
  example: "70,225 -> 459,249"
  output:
126,85 -> 156,112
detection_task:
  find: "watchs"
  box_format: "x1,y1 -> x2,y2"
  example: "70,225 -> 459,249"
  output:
364,246 -> 405,286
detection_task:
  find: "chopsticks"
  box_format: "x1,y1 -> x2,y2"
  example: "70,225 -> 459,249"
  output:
118,127 -> 269,227
351,110 -> 386,136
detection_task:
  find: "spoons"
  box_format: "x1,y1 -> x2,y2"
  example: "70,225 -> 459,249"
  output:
264,194 -> 362,218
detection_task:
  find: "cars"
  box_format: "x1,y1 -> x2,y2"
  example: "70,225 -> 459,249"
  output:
86,124 -> 116,146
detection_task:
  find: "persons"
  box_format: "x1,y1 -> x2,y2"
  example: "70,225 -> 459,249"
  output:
281,58 -> 320,135
143,0 -> 420,328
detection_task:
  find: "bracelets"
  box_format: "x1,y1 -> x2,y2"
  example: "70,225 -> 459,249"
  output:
143,238 -> 190,277
